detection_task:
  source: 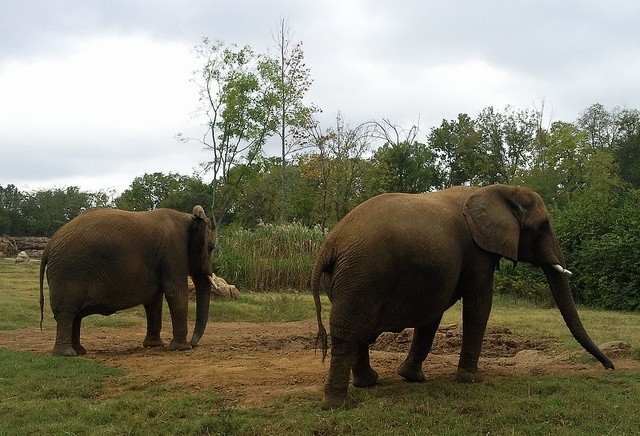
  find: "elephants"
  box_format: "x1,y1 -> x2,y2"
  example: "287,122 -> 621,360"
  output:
310,184 -> 616,412
38,204 -> 216,357
